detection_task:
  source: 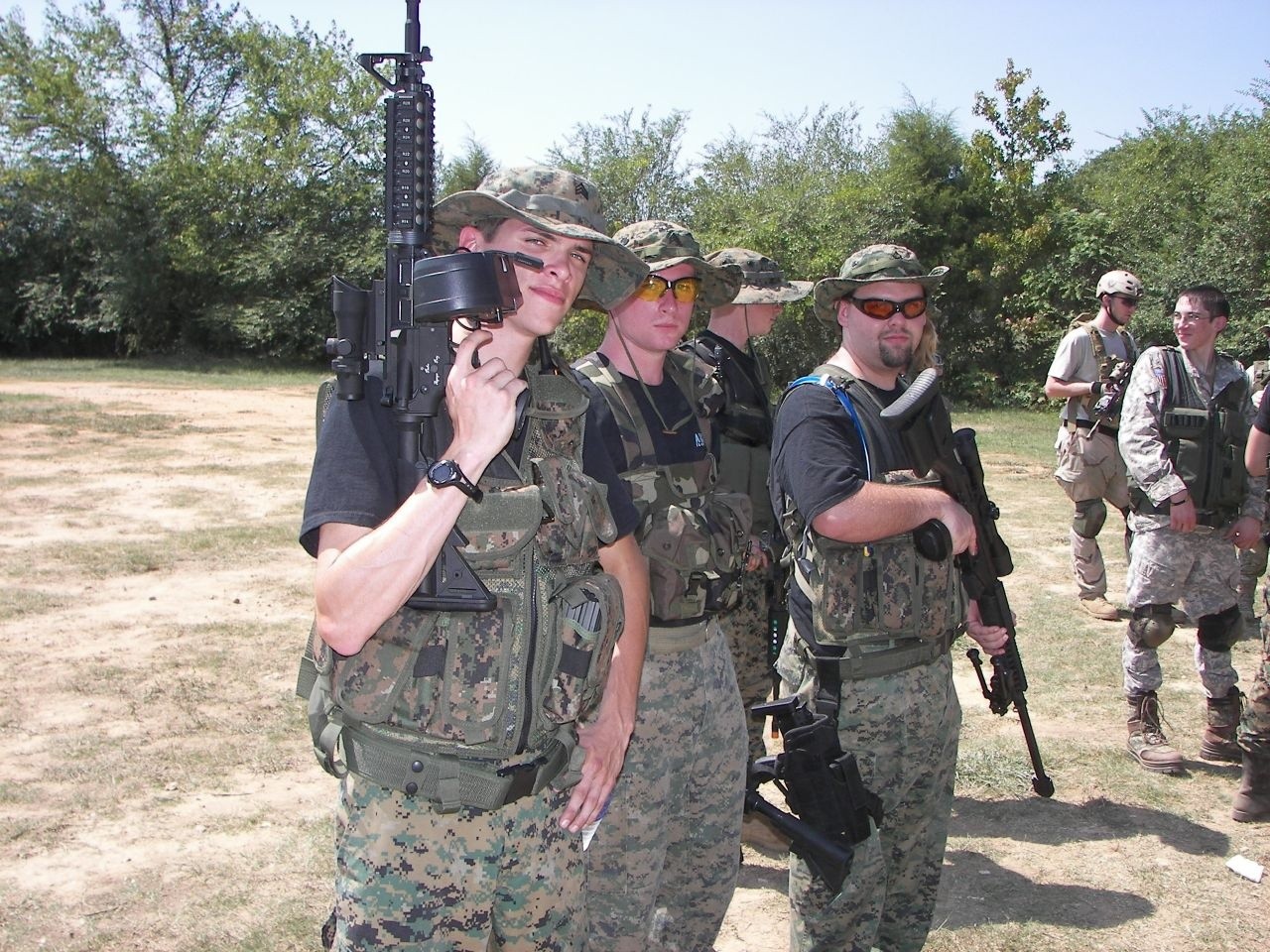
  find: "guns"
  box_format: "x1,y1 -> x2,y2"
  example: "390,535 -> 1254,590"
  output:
1086,361 -> 1133,441
880,367 -> 1054,797
760,572 -> 789,741
751,696 -> 813,736
325,0 -> 523,613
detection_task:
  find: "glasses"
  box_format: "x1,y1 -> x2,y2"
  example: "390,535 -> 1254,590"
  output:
1172,313 -> 1215,323
839,296 -> 927,321
632,273 -> 704,303
1110,297 -> 1139,307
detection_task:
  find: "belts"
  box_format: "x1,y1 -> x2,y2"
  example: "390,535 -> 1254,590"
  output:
798,623 -> 956,680
647,614 -> 719,652
1193,509 -> 1232,530
1063,419 -> 1118,440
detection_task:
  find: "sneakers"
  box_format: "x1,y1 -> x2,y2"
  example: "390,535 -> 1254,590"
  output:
1171,606 -> 1186,624
1078,595 -> 1119,618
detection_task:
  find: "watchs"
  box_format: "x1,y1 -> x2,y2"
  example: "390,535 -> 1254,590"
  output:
426,459 -> 484,504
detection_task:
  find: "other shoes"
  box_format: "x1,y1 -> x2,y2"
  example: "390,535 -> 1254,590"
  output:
739,807 -> 793,854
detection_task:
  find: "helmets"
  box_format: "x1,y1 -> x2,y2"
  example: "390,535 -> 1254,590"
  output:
1096,270 -> 1144,300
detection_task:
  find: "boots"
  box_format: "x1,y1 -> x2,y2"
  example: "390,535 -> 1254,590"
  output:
1239,579 -> 1259,620
1127,692 -> 1185,774
1199,685 -> 1243,763
1233,747 -> 1270,822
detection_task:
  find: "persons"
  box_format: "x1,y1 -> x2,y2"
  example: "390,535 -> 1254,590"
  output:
296,165 -> 1018,952
1043,270 -> 1270,824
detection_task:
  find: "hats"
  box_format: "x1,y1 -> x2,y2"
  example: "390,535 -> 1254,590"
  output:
813,244 -> 949,335
571,221 -> 745,314
701,249 -> 815,305
428,166 -> 650,309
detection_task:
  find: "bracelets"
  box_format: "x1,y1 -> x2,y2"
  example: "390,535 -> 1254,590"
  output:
1169,495 -> 1192,507
